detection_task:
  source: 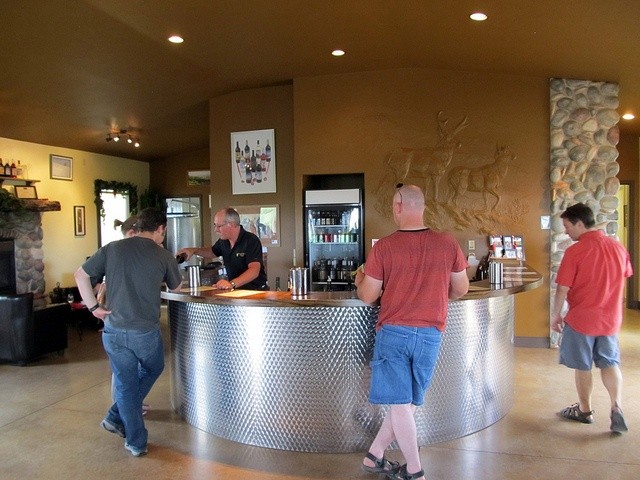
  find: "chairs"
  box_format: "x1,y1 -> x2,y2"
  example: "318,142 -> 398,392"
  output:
0,293 -> 71,366
54,286 -> 104,330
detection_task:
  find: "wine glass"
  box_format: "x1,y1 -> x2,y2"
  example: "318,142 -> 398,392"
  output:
68,292 -> 74,308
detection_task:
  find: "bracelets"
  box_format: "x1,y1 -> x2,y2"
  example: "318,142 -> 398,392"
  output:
229,280 -> 236,289
88,303 -> 99,312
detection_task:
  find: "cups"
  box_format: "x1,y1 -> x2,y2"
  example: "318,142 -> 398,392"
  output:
188,265 -> 201,288
291,268 -> 308,296
488,262 -> 505,284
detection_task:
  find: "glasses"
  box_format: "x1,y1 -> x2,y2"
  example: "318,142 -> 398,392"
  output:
213,223 -> 227,227
395,182 -> 406,204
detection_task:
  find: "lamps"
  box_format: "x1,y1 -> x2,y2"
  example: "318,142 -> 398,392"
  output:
127,135 -> 134,144
105,134 -> 113,142
113,133 -> 120,143
134,139 -> 139,148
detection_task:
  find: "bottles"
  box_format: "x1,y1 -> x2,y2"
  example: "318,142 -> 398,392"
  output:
175,253 -> 186,265
333,230 -> 355,243
0,158 -> 4,176
311,211 -> 339,226
17,160 -> 24,179
341,210 -> 350,225
313,258 -> 338,281
314,231 -> 331,243
4,160 -> 10,177
338,257 -> 358,281
10,160 -> 17,178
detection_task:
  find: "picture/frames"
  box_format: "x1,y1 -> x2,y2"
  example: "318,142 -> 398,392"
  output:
14,185 -> 37,199
223,204 -> 281,247
74,206 -> 86,236
185,169 -> 210,186
51,154 -> 73,181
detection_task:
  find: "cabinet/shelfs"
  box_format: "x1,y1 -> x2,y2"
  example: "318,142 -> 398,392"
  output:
0,176 -> 40,186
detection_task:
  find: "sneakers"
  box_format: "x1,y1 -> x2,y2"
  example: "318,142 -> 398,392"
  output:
101,416 -> 126,438
125,438 -> 148,457
142,403 -> 151,416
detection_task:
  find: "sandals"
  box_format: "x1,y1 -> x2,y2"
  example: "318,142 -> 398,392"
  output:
360,452 -> 400,474
610,401 -> 628,431
385,463 -> 424,480
560,402 -> 594,423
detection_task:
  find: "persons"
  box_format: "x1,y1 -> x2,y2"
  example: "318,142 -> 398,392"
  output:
93,214 -> 150,416
355,185 -> 471,480
550,202 -> 634,434
176,207 -> 270,291
73,208 -> 183,459
